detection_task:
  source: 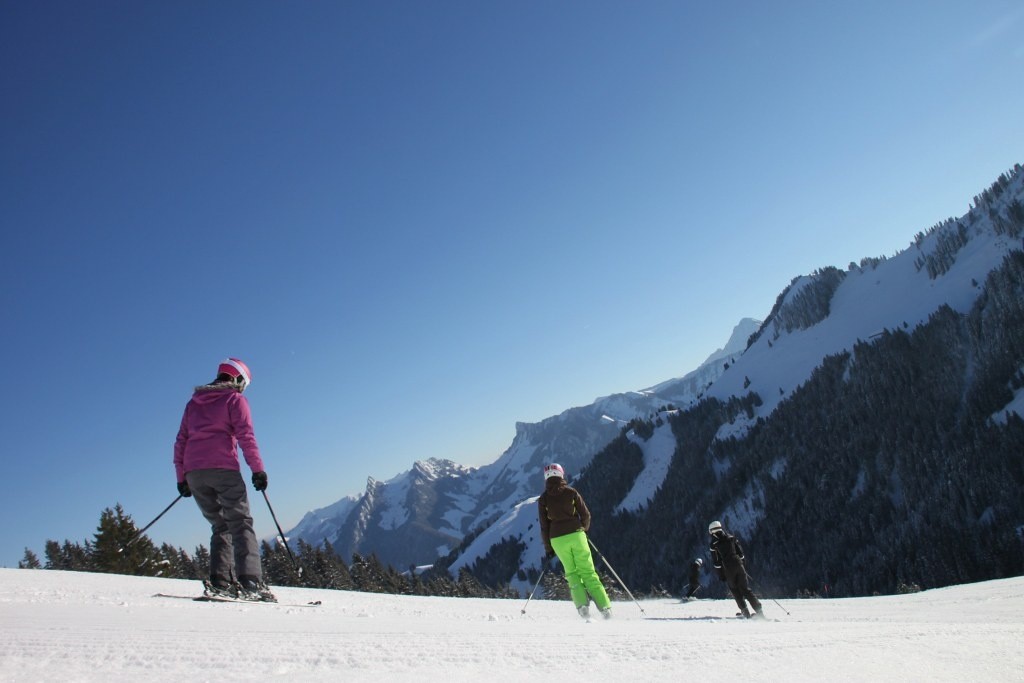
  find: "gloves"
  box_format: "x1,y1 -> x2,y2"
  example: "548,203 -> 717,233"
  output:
546,550 -> 555,559
177,481 -> 192,497
252,472 -> 268,492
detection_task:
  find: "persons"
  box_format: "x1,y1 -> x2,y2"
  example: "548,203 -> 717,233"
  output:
173,358 -> 276,601
685,558 -> 703,600
709,521 -> 763,619
539,463 -> 613,622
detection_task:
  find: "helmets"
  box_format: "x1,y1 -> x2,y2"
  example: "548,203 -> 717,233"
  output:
544,463 -> 564,480
709,521 -> 722,535
695,558 -> 703,566
218,358 -> 251,385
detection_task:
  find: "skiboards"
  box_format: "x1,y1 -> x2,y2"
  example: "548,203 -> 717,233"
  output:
151,592 -> 323,608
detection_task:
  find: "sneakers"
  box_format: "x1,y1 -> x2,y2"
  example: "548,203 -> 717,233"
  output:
578,605 -> 590,619
601,609 -> 612,620
212,580 -> 244,599
752,613 -> 764,618
237,576 -> 271,597
736,612 -> 750,618
